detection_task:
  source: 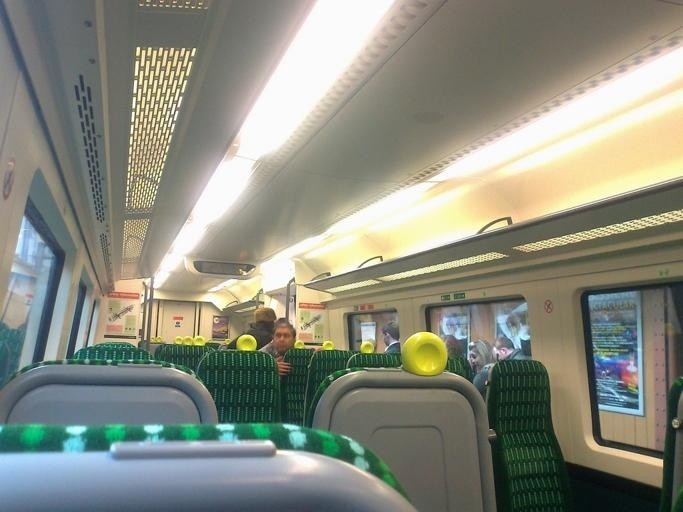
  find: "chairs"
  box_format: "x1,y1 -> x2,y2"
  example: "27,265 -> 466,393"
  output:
1,421 -> 414,512
278,339 -> 573,511
0,339 -> 282,425
658,377 -> 683,512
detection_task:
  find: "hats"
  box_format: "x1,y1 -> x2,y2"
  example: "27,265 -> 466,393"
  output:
255,307 -> 276,321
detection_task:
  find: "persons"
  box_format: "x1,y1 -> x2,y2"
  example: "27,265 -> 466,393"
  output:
381,321 -> 400,354
441,335 -> 532,402
227,307 -> 296,376
